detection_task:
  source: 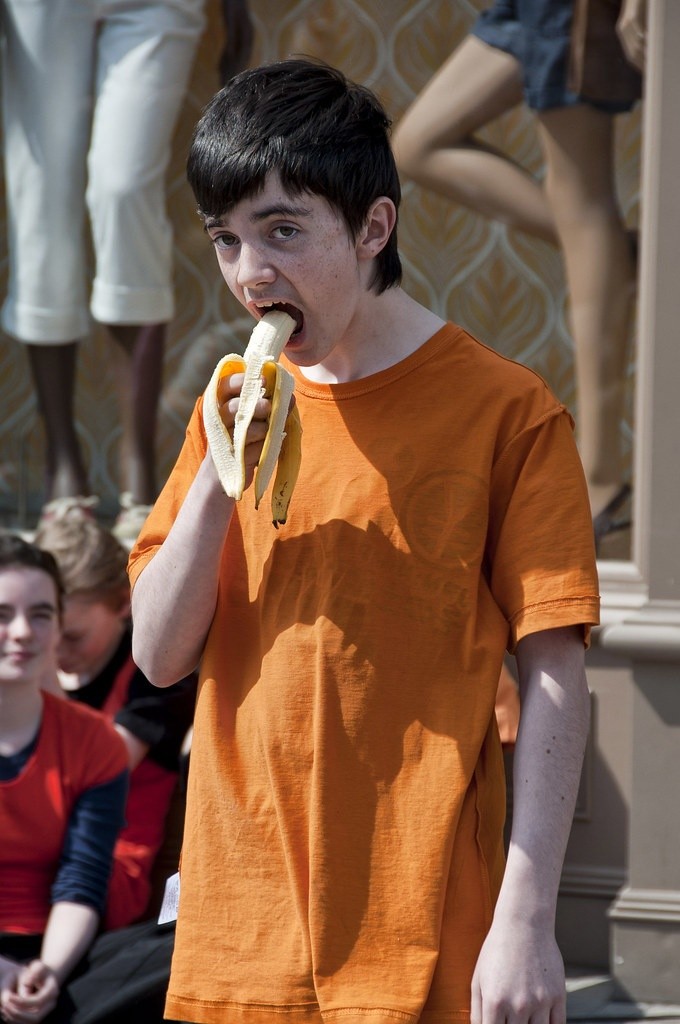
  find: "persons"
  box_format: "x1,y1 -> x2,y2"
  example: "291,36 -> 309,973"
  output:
392,0 -> 646,543
29,495 -> 200,929
0,1 -> 256,543
0,535 -> 179,1024
128,52 -> 601,1024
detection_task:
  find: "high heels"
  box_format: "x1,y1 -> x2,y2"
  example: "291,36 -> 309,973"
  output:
590,482 -> 635,559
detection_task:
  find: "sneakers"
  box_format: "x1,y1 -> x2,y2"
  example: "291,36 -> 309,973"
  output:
109,503 -> 153,556
33,494 -> 100,537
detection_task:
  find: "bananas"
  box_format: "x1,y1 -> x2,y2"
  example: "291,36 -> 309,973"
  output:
202,311 -> 301,523
